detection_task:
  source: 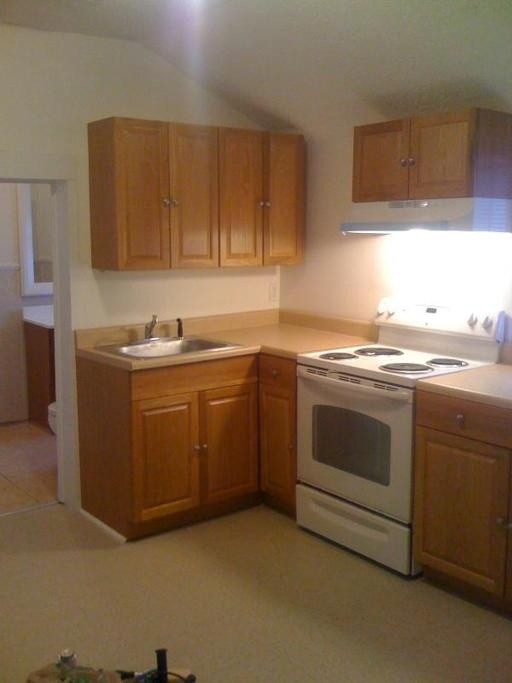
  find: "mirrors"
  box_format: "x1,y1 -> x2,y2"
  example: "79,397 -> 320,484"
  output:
17,182 -> 53,297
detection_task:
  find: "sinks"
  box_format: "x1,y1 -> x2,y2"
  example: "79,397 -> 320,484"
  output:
93,335 -> 246,362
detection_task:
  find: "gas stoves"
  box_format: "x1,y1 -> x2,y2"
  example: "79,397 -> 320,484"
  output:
296,343 -> 495,389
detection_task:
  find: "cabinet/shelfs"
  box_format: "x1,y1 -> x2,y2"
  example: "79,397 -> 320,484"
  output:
259,352 -> 298,520
23,322 -> 54,421
221,126 -> 306,266
411,386 -> 512,615
87,117 -> 222,272
74,355 -> 267,544
351,109 -> 511,203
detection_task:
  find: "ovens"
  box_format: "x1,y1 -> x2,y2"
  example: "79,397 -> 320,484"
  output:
296,364 -> 415,575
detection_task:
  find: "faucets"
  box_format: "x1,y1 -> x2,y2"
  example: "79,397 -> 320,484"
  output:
145,315 -> 158,338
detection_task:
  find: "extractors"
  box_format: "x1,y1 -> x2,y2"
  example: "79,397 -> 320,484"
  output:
340,197 -> 512,235
